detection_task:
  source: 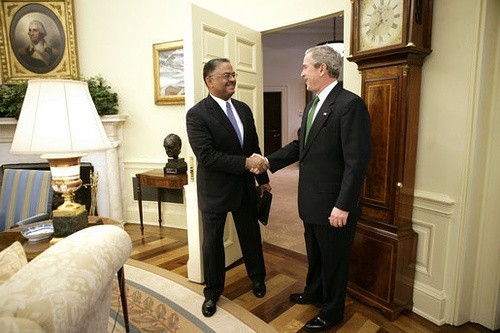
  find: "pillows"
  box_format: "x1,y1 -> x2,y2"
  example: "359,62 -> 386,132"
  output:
0,240 -> 27,287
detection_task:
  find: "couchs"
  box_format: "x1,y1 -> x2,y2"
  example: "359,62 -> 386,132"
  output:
0,225 -> 133,333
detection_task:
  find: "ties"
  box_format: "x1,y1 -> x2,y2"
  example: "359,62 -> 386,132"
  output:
304,96 -> 320,149
227,102 -> 243,147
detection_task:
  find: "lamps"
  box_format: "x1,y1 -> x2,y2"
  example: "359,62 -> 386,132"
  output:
315,17 -> 345,57
9,79 -> 112,237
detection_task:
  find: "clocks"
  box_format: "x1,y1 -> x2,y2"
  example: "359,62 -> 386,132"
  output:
340,0 -> 435,321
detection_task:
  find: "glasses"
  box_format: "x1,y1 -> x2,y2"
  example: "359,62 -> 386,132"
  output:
209,73 -> 238,80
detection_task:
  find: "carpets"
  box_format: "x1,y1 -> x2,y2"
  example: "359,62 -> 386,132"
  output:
52,237 -> 279,333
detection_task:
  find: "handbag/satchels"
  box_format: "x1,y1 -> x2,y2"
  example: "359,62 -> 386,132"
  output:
256,186 -> 272,225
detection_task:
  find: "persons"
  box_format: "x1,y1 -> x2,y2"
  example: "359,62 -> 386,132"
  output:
249,46 -> 370,333
186,58 -> 271,319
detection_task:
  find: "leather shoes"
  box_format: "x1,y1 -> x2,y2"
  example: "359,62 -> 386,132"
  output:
202,295 -> 219,316
252,279 -> 266,297
304,313 -> 344,331
290,293 -> 324,306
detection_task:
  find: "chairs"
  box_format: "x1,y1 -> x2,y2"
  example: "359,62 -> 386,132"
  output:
0,168 -> 54,231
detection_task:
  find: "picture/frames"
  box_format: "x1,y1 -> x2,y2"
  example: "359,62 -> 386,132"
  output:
153,39 -> 187,105
0,0 -> 81,86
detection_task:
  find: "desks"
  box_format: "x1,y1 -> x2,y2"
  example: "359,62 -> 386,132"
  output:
133,168 -> 188,237
0,215 -> 139,332
